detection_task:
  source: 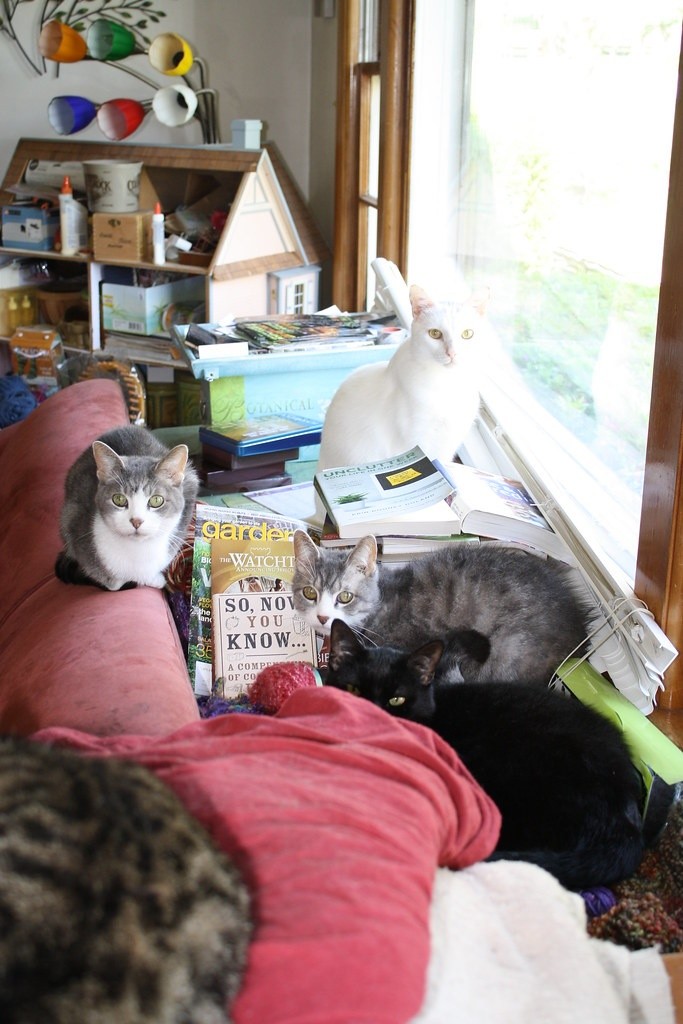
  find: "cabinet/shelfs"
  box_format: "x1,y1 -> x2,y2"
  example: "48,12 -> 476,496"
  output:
0,136 -> 324,429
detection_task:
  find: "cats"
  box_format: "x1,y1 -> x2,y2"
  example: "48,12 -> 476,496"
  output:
313,278 -> 495,530
54,425 -> 201,593
0,736 -> 259,1023
290,524 -> 650,898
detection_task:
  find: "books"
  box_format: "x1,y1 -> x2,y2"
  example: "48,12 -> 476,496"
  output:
103,332 -> 177,361
187,446 -> 578,704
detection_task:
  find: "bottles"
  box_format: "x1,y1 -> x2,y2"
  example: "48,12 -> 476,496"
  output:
58,178 -> 89,256
9,298 -> 19,329
21,295 -> 31,325
152,203 -> 165,264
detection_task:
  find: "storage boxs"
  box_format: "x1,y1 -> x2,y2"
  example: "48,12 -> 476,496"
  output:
92,212 -> 151,264
1,203 -> 61,250
103,276 -> 207,335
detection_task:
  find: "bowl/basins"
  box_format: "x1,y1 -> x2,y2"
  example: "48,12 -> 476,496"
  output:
37,281 -> 80,323
82,158 -> 144,212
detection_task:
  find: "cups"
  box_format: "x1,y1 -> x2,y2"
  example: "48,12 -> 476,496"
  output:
177,376 -> 200,425
147,383 -> 177,427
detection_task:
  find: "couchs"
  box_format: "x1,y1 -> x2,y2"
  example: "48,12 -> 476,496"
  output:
1,379 -> 204,735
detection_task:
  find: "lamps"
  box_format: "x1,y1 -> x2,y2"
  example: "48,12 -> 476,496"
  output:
36,20 -> 220,144
